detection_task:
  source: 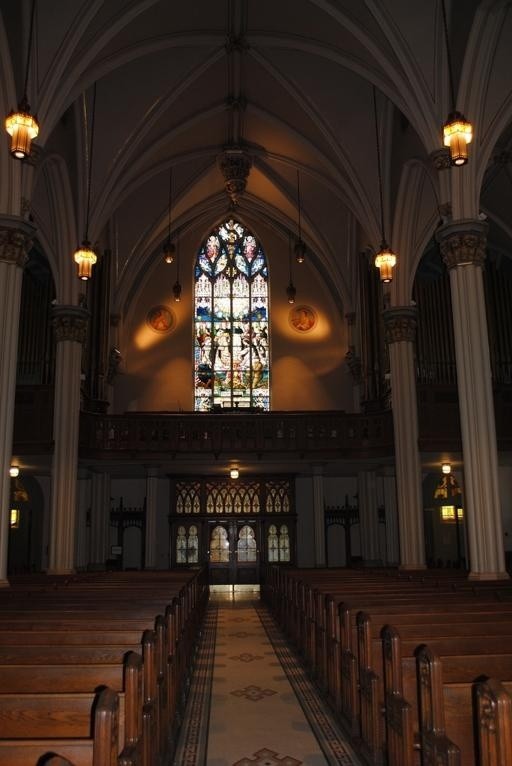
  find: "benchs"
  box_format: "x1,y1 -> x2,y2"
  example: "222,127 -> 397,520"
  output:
262,566 -> 511,766
0,566 -> 210,764
160,166 -> 182,303
285,164 -> 308,306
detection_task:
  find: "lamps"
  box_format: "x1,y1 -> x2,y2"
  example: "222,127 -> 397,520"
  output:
437,1 -> 473,166
73,80 -> 103,279
8,502 -> 23,529
371,81 -> 397,279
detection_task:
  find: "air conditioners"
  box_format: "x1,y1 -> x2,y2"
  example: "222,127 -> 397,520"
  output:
2,0 -> 38,158
